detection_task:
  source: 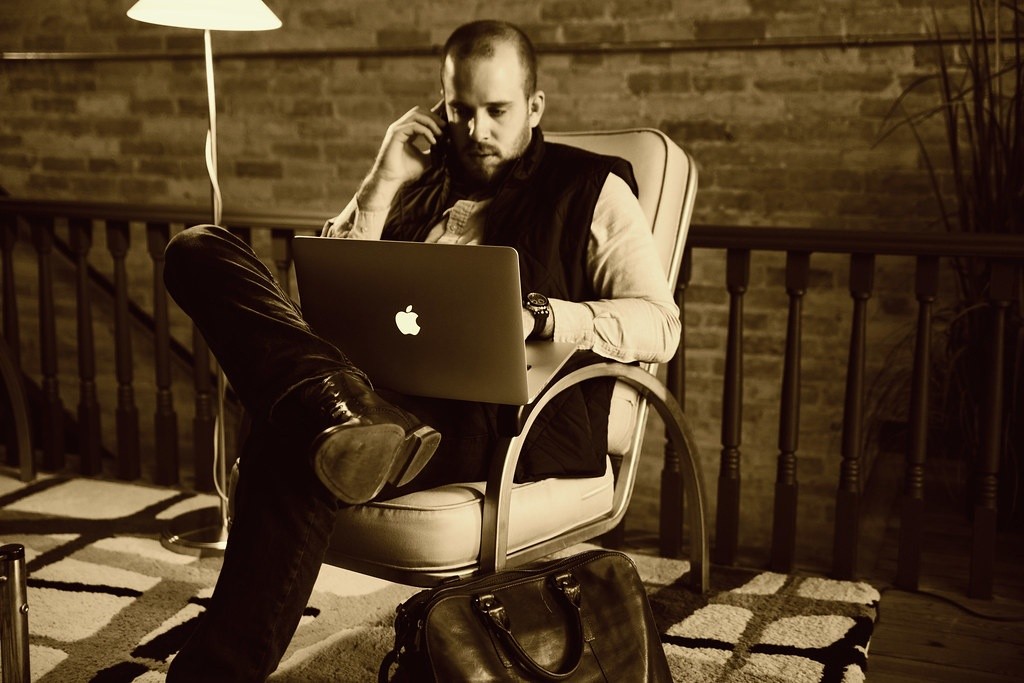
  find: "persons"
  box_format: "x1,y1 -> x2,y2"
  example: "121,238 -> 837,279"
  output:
161,19 -> 683,683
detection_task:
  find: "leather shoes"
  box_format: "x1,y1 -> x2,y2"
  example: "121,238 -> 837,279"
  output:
300,371 -> 444,505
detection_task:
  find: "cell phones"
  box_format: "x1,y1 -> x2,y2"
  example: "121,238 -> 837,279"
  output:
428,98 -> 450,167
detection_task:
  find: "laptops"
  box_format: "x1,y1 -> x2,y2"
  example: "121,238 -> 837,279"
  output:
295,235 -> 579,405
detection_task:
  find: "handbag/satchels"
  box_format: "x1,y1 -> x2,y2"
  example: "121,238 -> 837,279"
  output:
382,543 -> 673,682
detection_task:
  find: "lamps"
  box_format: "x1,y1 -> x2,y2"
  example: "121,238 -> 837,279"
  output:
127,0 -> 284,558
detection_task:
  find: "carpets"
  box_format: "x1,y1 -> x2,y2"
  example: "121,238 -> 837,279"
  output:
1,470 -> 881,683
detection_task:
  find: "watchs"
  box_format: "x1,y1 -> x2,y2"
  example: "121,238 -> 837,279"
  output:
522,292 -> 549,337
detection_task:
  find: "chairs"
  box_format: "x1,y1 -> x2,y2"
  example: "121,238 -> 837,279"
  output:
228,127 -> 711,597
